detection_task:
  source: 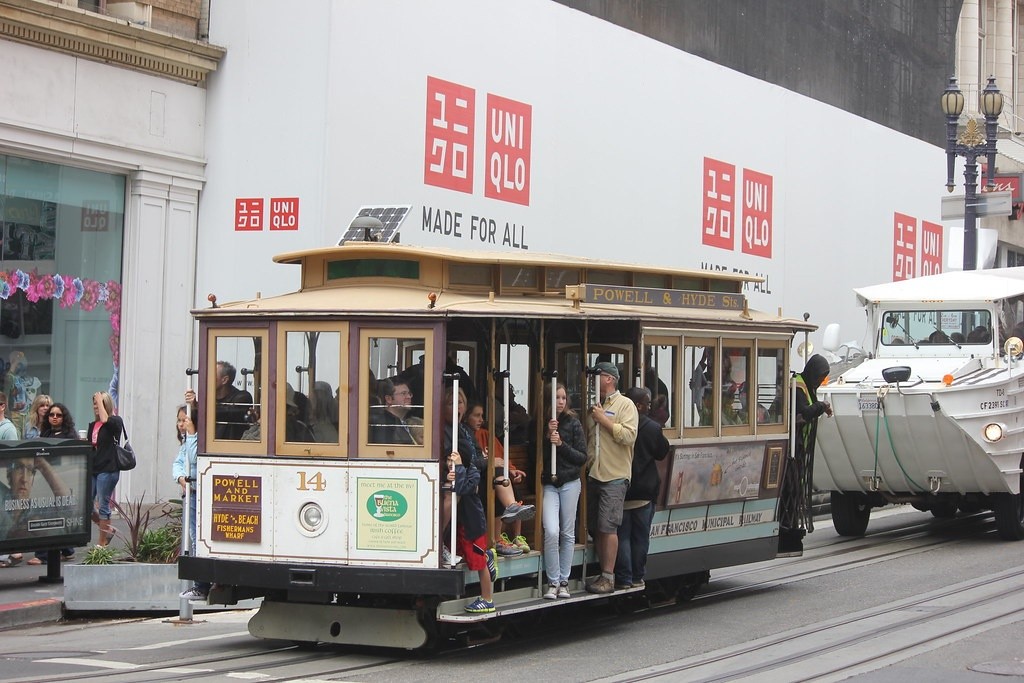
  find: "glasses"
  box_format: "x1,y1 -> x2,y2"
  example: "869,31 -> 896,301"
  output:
639,401 -> 652,406
392,391 -> 413,399
49,413 -> 64,418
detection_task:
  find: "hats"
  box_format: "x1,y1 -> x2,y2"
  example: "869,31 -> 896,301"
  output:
0,392 -> 7,405
586,362 -> 620,379
738,382 -> 746,392
285,382 -> 297,408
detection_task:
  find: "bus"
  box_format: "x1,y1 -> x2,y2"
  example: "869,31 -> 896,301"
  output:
178,205 -> 819,657
812,263 -> 1024,538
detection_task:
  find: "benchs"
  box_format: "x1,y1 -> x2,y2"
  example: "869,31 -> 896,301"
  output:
500,446 -> 536,544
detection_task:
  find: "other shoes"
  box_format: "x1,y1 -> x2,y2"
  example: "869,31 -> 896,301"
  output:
105,528 -> 116,545
27,557 -> 43,565
587,535 -> 593,542
543,582 -> 559,600
615,582 -> 631,589
441,545 -> 463,565
631,579 -> 645,587
558,581 -> 571,598
60,552 -> 77,561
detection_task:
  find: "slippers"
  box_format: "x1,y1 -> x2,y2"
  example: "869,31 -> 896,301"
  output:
1,556 -> 23,568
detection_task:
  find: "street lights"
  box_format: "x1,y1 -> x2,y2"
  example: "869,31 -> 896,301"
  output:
941,73 -> 1004,336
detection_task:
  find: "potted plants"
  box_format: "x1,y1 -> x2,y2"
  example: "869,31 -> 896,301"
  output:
63,490 -> 195,610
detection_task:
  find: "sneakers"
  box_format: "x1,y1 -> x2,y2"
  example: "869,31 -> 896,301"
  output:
501,501 -> 537,524
586,576 -> 615,593
464,596 -> 495,612
513,536 -> 530,553
486,548 -> 498,582
495,535 -> 522,556
500,533 -> 519,550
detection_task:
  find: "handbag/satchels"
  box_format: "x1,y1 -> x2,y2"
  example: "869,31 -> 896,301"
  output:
113,424 -> 136,471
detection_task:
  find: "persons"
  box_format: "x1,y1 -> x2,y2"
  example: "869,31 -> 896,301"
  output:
185,361 -> 424,445
541,383 -> 586,600
768,354 -> 832,529
617,387 -> 669,588
24,395 -> 54,440
173,405 -> 198,557
444,386 -> 536,524
460,400 -> 530,558
446,439 -> 498,613
4,349 -> 43,433
495,380 -> 535,448
586,362 -> 638,594
689,346 -> 744,426
0,456 -> 77,540
0,392 -> 18,441
737,381 -> 770,424
39,403 -> 80,438
890,321 -> 1024,345
87,390 -> 123,547
